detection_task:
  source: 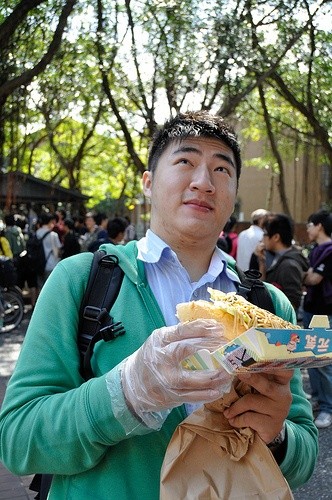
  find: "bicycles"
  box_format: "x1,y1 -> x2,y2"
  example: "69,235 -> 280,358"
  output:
0,287 -> 25,332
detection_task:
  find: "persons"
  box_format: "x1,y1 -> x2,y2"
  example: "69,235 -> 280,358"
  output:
254,212 -> 311,311
0,207 -> 135,311
301,210 -> 332,428
0,110 -> 318,500
235,208 -> 268,273
216,216 -> 238,261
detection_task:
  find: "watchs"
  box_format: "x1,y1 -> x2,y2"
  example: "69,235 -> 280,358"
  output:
266,421 -> 287,448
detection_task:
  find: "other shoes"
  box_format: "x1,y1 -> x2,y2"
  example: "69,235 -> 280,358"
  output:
314,410 -> 332,428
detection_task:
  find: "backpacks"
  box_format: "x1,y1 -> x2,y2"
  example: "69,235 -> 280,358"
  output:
27,231 -> 54,274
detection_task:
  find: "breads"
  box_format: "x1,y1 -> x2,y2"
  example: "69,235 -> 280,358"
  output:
176,288 -> 302,341
